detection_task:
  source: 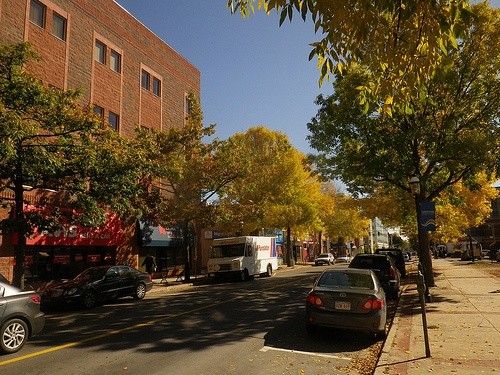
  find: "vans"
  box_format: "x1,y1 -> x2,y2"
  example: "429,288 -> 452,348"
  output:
207,235 -> 278,282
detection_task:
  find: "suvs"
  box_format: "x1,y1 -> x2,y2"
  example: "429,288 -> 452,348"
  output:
374,248 -> 406,278
347,252 -> 401,300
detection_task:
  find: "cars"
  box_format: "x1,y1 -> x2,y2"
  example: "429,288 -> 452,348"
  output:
402,252 -> 409,262
0,273 -> 46,355
43,264 -> 153,310
337,255 -> 351,263
306,268 -> 387,340
315,252 -> 336,265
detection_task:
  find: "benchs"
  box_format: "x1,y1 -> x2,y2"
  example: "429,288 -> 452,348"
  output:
159,265 -> 185,284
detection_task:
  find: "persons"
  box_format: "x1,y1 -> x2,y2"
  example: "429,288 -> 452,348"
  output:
141,254 -> 158,278
